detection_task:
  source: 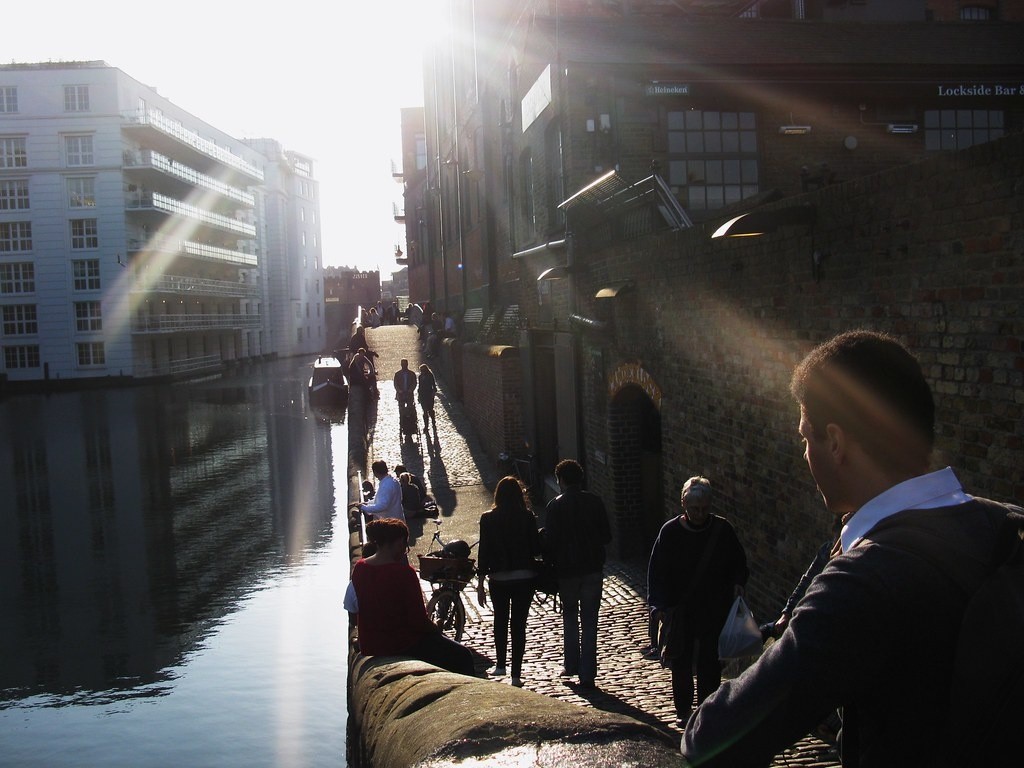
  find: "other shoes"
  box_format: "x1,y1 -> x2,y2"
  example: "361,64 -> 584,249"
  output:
561,670 -> 579,676
676,716 -> 689,725
512,678 -> 525,686
491,666 -> 506,675
641,645 -> 660,659
580,679 -> 595,689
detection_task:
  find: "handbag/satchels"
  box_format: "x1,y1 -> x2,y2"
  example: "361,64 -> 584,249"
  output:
533,556 -> 563,614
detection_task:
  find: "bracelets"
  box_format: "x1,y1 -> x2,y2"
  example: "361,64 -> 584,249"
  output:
476,587 -> 484,592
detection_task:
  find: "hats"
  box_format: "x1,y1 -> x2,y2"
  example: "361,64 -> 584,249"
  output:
395,466 -> 407,472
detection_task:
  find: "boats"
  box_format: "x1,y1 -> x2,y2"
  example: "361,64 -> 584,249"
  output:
307,355 -> 348,404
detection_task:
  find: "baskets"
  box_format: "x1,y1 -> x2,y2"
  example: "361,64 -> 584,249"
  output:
420,558 -> 476,583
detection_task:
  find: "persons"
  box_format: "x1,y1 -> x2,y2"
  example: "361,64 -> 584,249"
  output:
417,303 -> 456,359
544,461 -> 612,688
355,460 -> 426,525
353,518 -> 475,677
400,303 -> 412,324
360,300 -> 399,328
418,364 -> 437,431
680,332 -> 1024,768
349,327 -> 379,393
648,477 -> 746,727
394,359 -> 417,431
759,512 -> 842,736
344,543 -> 375,626
476,476 -> 546,687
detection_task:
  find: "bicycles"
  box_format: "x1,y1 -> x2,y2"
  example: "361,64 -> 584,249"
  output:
415,518 -> 481,644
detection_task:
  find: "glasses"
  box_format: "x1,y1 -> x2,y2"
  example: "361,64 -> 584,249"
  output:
687,505 -> 713,513
404,546 -> 409,555
